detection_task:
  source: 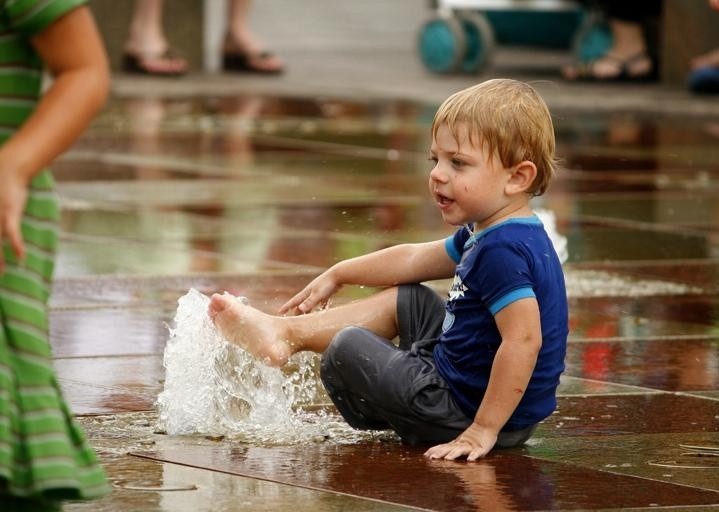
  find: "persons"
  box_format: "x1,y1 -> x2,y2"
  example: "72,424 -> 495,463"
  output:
118,0 -> 287,78
1,0 -> 118,511
320,441 -> 557,512
206,73 -> 570,465
558,0 -> 665,84
682,0 -> 718,98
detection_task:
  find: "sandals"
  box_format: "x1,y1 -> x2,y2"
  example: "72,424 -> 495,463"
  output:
224,30 -> 283,74
122,43 -> 187,76
562,52 -> 654,81
